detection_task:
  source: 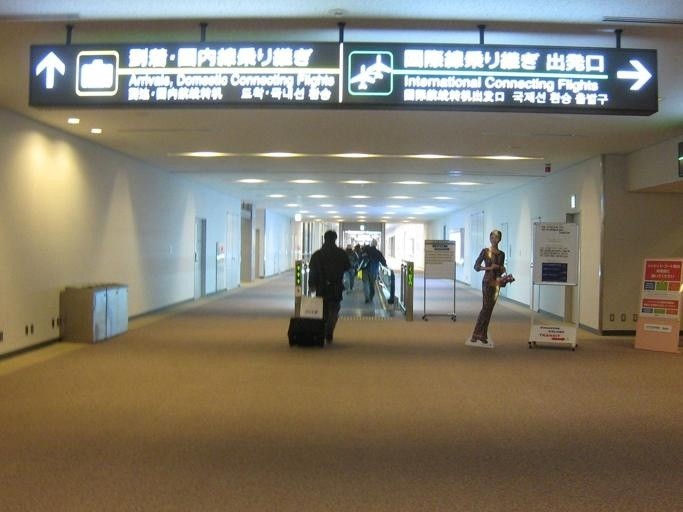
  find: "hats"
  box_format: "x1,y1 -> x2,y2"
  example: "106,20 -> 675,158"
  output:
371,239 -> 377,246
347,244 -> 351,249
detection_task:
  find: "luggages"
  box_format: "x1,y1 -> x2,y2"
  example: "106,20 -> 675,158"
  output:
287,289 -> 327,349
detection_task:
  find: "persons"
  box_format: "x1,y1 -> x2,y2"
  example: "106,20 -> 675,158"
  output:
309,231 -> 350,344
472,230 -> 507,344
344,239 -> 387,304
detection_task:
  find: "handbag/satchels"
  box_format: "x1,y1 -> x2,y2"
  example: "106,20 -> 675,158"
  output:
299,289 -> 324,320
359,247 -> 369,269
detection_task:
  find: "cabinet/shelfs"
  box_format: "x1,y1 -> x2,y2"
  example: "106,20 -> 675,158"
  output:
59,283 -> 128,344
